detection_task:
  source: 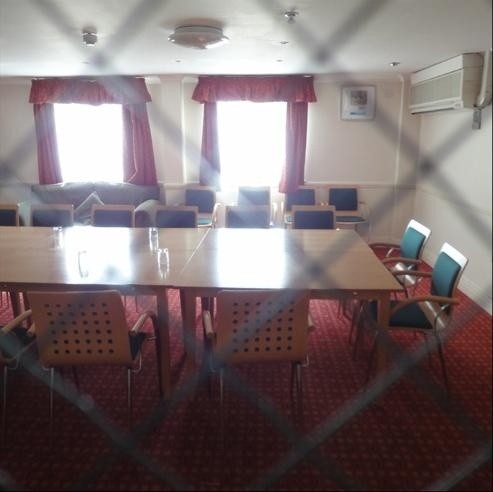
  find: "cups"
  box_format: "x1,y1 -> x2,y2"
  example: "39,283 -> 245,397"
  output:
52,225 -> 62,239
157,248 -> 169,265
157,265 -> 170,280
146,225 -> 159,240
78,264 -> 88,277
77,250 -> 87,264
150,239 -> 160,252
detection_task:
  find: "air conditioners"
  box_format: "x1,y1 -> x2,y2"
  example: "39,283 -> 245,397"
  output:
409,47 -> 492,114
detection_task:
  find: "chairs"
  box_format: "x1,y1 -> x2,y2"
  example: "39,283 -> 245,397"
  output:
205,286 -> 317,415
30,281 -> 161,425
340,219 -> 432,341
346,242 -> 469,394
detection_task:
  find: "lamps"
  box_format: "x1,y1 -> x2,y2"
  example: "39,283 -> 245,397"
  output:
168,25 -> 229,50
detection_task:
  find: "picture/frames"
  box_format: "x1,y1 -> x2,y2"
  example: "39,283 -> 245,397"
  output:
340,85 -> 376,121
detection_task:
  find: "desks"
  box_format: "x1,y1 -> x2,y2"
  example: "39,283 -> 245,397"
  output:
1,224 -> 405,393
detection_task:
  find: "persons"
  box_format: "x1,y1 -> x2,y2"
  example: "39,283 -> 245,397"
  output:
350,90 -> 367,115
